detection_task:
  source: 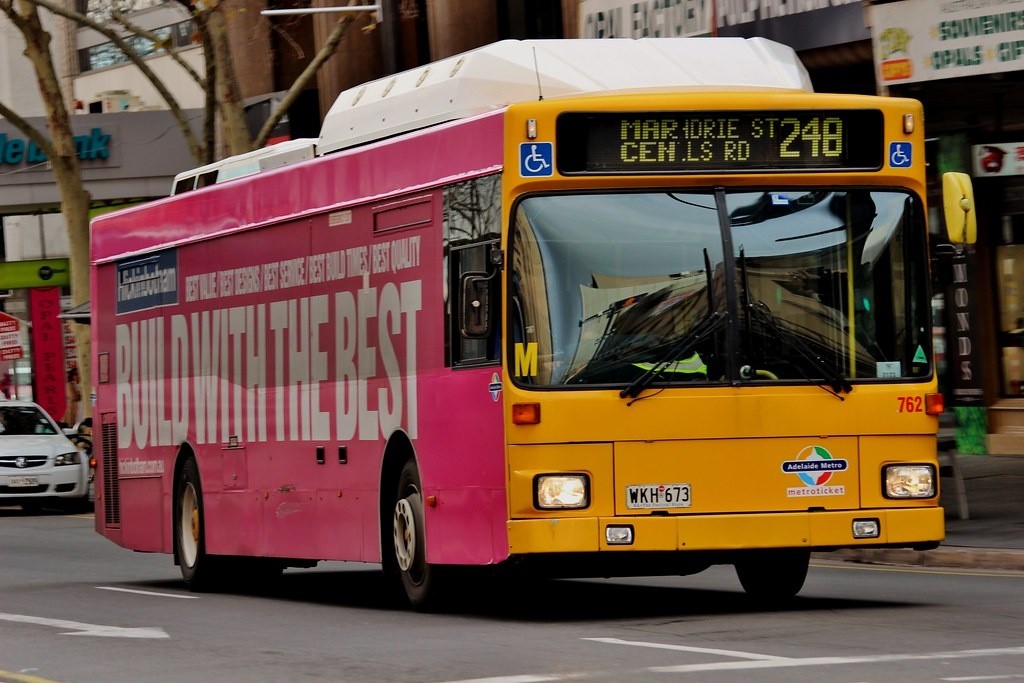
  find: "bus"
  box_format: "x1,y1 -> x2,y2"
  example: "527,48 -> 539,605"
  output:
86,35 -> 980,614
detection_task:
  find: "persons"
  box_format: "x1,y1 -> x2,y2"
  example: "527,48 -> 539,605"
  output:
65,368 -> 83,428
0,373 -> 13,401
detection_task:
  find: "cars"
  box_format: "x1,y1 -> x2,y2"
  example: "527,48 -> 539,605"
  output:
0,397 -> 88,517
